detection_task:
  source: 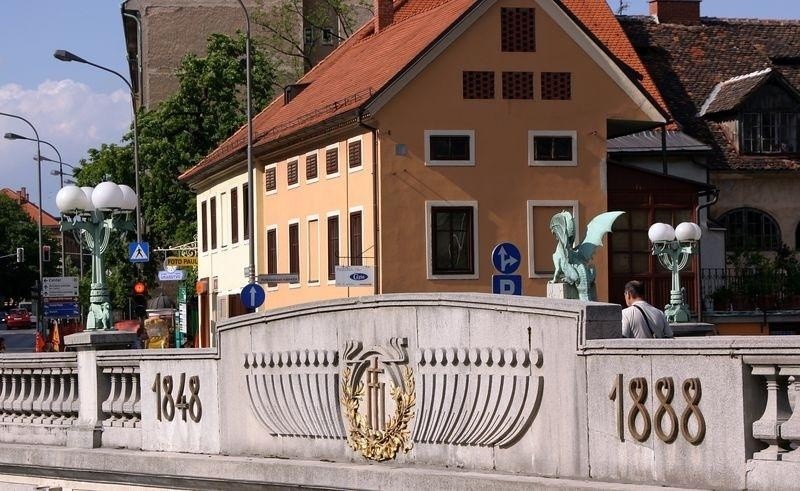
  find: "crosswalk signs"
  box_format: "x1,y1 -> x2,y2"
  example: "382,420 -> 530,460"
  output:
127,241 -> 150,265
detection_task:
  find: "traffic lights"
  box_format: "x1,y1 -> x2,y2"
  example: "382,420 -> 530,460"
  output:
131,276 -> 147,318
42,245 -> 51,262
16,248 -> 24,262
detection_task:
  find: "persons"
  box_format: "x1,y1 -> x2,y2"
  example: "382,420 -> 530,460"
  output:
0,335 -> 7,354
620,279 -> 675,339
179,333 -> 196,348
133,326 -> 149,349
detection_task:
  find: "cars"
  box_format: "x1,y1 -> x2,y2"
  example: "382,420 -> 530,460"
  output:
6,308 -> 32,329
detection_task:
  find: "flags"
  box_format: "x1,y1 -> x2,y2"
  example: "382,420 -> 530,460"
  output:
49,321 -> 63,353
34,328 -> 45,354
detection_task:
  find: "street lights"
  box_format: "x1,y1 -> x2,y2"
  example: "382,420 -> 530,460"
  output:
647,221 -> 702,323
4,132 -> 85,278
53,48 -> 143,272
